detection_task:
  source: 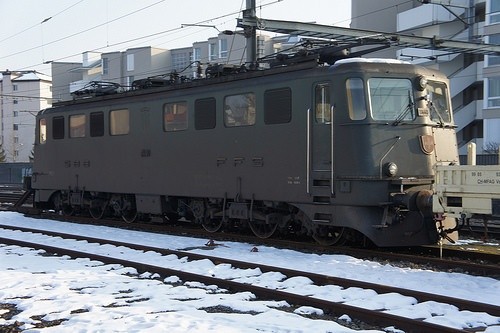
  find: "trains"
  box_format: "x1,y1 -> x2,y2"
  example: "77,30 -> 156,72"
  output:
29,33 -> 462,260
